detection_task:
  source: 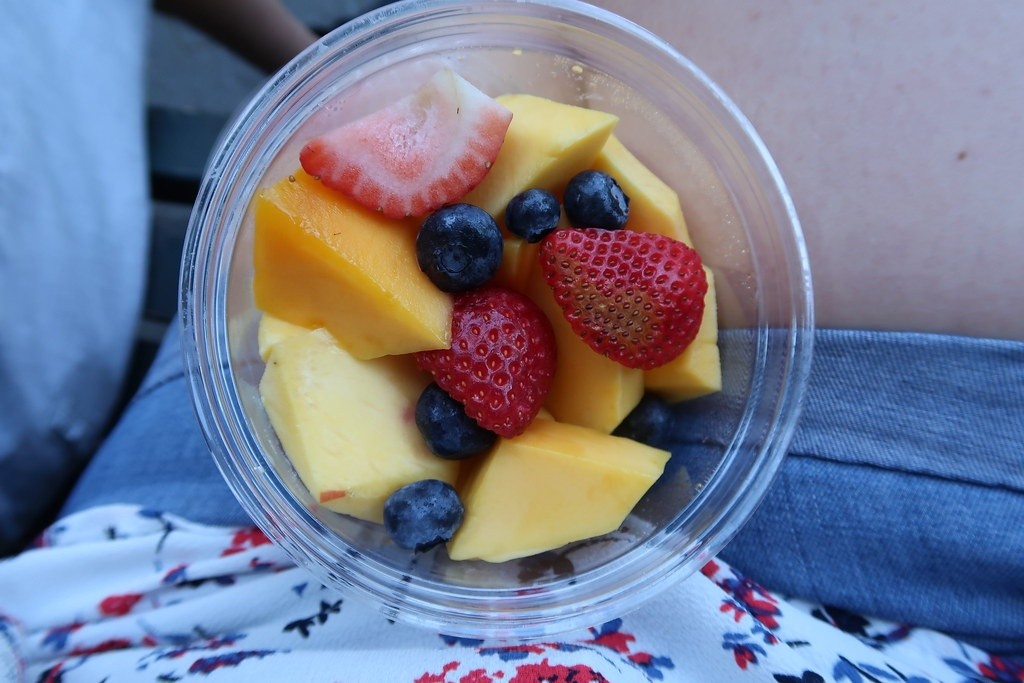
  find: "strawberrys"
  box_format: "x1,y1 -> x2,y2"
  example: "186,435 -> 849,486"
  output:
298,68 -> 513,218
411,285 -> 555,439
538,228 -> 708,370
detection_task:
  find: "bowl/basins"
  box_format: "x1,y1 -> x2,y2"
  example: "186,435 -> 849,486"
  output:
177,0 -> 815,637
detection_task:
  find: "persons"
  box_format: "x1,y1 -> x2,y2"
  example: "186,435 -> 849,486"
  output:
1,0 -> 320,558
1,1 -> 1023,683
